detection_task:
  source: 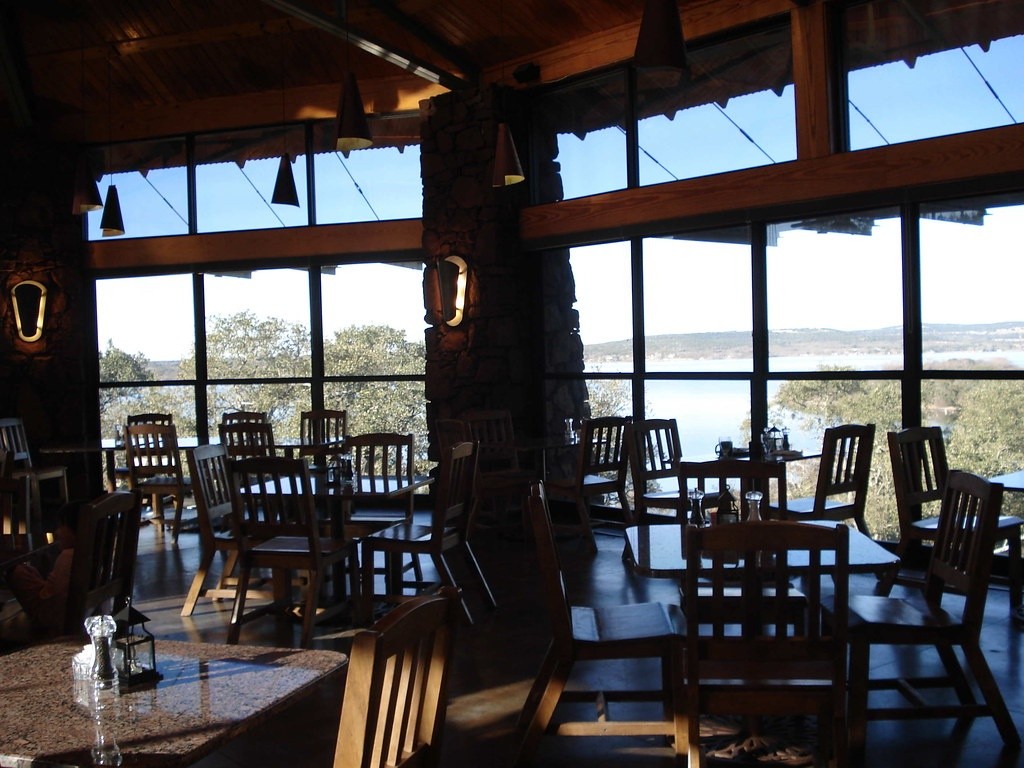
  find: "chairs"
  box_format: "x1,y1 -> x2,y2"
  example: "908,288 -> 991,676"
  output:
0,410 -> 1024,768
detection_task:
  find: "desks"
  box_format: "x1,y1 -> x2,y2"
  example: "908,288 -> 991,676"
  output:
986,470 -> 1024,625
102,438 -> 162,493
39,442 -> 108,506
0,636 -> 349,768
133,436 -> 246,535
661,446 -> 822,509
232,436 -> 345,459
238,475 -> 435,628
623,519 -> 902,768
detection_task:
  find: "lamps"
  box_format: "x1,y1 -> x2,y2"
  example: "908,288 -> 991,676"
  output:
335,0 -> 374,151
270,23 -> 300,207
11,279 -> 50,343
113,596 -> 165,690
632,0 -> 688,74
437,251 -> 470,330
74,38 -> 102,212
716,484 -> 739,525
490,0 -> 525,190
328,454 -> 346,488
99,62 -> 125,236
767,425 -> 784,450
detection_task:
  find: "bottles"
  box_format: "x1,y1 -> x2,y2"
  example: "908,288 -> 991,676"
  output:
761,428 -> 772,459
784,433 -> 790,450
85,615 -> 119,688
688,488 -> 706,528
745,491 -> 763,521
114,424 -> 121,446
565,418 -> 574,444
575,432 -> 582,444
341,455 -> 353,482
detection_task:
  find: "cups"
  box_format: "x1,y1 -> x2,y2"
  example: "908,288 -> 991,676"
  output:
719,436 -> 731,448
72,644 -> 123,680
715,441 -> 733,458
